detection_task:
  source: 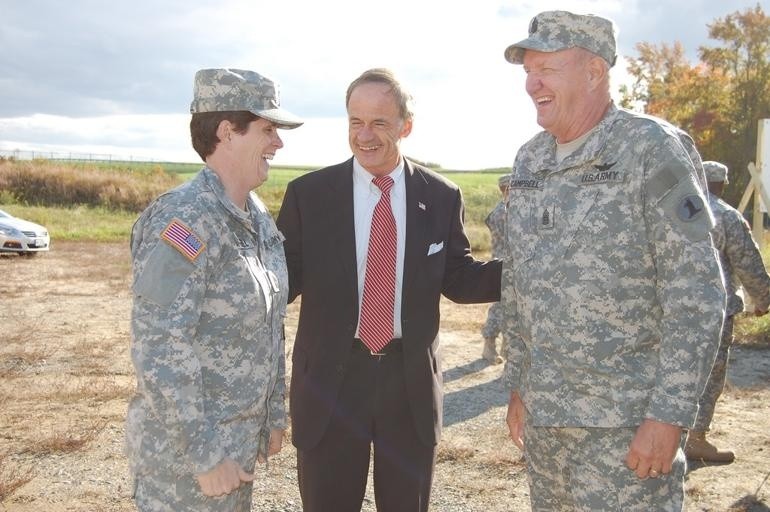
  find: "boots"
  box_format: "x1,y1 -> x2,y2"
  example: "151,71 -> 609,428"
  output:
684,431 -> 734,463
482,335 -> 503,364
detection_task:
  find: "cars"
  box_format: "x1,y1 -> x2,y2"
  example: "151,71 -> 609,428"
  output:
1,205 -> 51,257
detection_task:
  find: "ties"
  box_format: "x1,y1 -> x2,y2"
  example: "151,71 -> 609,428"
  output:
358,177 -> 397,354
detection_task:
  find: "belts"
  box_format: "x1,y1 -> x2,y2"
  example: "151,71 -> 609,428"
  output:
352,338 -> 402,359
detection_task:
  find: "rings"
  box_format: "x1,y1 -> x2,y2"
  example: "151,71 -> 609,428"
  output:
650,466 -> 662,477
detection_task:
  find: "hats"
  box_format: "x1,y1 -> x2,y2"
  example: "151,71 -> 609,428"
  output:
504,10 -> 616,65
192,68 -> 303,130
703,160 -> 729,185
498,175 -> 510,187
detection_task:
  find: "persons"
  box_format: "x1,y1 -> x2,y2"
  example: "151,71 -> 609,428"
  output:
127,65 -> 291,508
484,10 -> 729,512
275,66 -> 506,512
681,157 -> 770,464
478,175 -> 514,367
